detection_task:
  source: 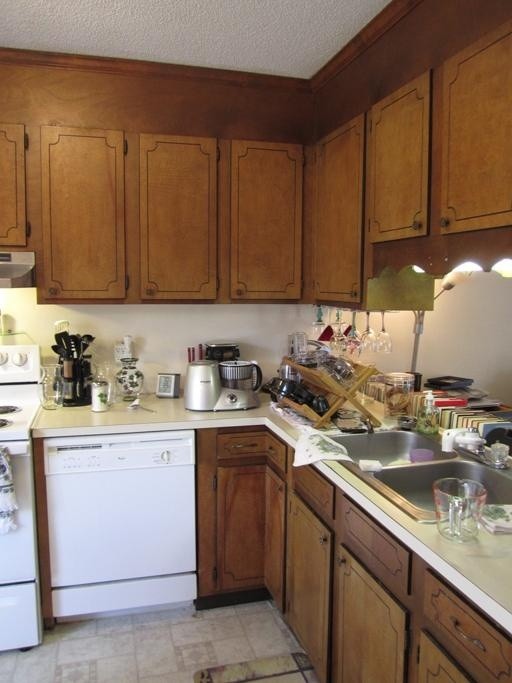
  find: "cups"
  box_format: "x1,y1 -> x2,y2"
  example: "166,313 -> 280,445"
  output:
36,362 -> 68,411
431,476 -> 488,543
489,441 -> 509,467
382,371 -> 415,421
409,447 -> 433,462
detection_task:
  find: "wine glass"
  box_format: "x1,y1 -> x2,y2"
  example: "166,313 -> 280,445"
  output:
311,302 -> 393,354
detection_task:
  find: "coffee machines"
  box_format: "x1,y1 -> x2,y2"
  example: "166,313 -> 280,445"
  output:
182,340 -> 264,412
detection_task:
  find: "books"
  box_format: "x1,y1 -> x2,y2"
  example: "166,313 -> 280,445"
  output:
351,375 -> 512,450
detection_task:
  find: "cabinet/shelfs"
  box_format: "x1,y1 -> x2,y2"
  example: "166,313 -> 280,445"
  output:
214,427 -> 287,625
415,566 -> 511,683
284,445 -> 412,683
305,110 -> 436,312
0,122 -> 31,250
361,16 -> 511,244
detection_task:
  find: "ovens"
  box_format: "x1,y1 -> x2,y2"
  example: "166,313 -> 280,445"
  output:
0,437 -> 44,660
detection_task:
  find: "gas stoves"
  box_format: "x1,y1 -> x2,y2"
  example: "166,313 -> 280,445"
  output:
0,382 -> 41,445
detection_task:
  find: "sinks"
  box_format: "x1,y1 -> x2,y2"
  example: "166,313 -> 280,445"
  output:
325,430 -> 457,469
373,460 -> 511,524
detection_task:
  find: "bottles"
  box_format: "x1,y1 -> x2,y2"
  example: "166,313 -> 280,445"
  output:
89,360 -> 115,414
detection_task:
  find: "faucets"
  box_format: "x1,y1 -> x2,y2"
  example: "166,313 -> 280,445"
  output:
441,427 -> 486,453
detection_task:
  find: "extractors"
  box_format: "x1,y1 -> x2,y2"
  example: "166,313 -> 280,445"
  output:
0,250 -> 36,285
